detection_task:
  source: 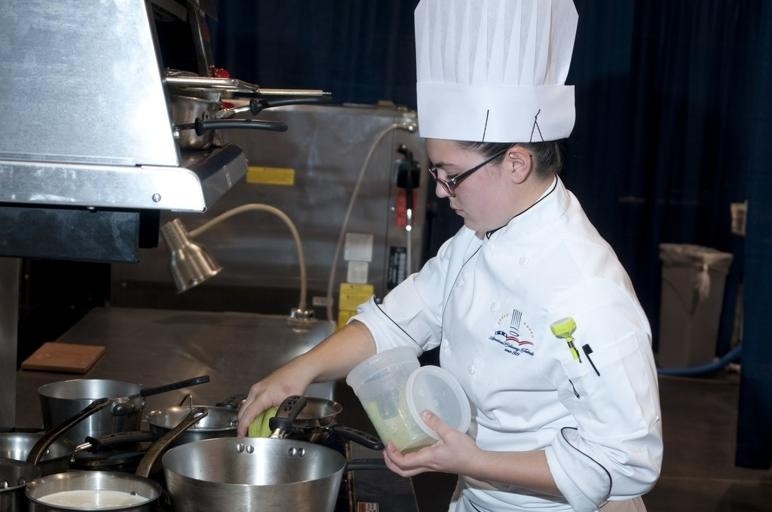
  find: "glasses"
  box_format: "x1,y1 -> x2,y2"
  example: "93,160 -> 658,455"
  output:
429,151 -> 508,196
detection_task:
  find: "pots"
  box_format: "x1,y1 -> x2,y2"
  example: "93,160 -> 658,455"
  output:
270,395 -> 384,455
2,399 -> 111,510
2,430 -> 73,479
163,396 -> 348,512
24,409 -> 208,512
40,373 -> 211,449
167,93 -> 335,153
147,403 -> 249,475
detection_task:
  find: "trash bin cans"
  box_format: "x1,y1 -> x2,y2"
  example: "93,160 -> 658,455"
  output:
655,242 -> 734,371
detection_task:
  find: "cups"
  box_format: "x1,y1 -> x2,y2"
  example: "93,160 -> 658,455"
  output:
345,347 -> 442,453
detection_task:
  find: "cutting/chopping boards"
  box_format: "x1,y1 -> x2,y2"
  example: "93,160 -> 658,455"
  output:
23,342 -> 106,374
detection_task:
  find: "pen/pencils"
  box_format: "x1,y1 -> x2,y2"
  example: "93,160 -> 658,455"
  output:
583,344 -> 604,376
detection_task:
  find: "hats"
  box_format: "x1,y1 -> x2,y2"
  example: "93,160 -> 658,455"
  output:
414,2 -> 579,142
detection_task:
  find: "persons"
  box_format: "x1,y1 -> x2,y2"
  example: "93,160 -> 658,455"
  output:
236,137 -> 666,512
